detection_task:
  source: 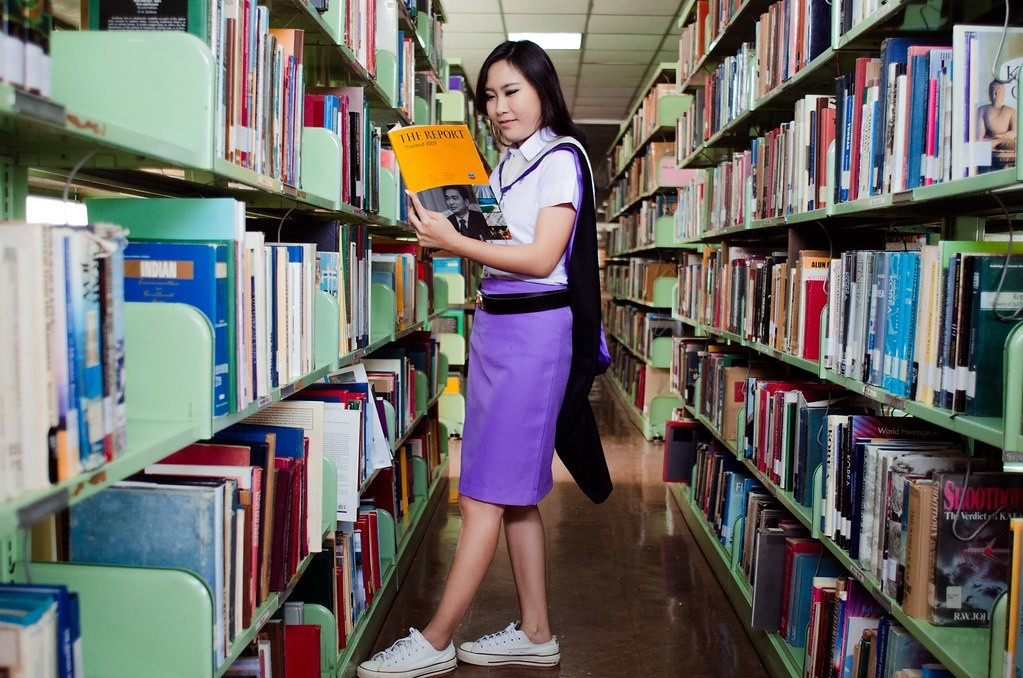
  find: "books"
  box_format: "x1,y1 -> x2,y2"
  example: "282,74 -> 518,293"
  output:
387,121 -> 512,243
0,0 -> 498,230
0,193 -> 474,502
606,192 -> 678,419
606,0 -> 744,218
663,0 -> 1022,678
0,317 -> 443,678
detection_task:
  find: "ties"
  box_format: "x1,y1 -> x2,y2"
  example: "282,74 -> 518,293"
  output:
458,219 -> 468,236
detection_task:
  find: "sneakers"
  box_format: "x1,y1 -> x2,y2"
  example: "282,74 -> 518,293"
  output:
356,626 -> 457,678
457,621 -> 560,666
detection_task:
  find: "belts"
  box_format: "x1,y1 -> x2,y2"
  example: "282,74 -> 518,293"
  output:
473,281 -> 571,316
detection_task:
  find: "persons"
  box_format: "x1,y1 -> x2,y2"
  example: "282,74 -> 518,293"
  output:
355,40 -> 611,678
441,185 -> 489,241
976,77 -> 1017,151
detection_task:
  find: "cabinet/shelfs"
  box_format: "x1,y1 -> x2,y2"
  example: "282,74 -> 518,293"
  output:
595,0 -> 1023,678
0,0 -> 499,678
